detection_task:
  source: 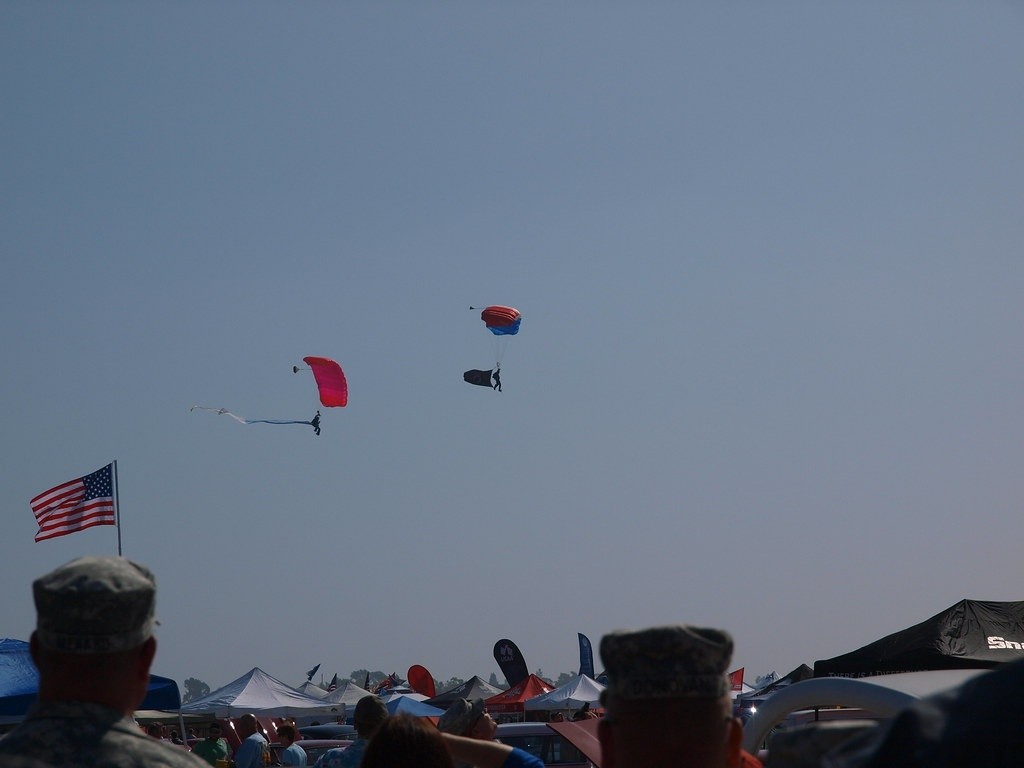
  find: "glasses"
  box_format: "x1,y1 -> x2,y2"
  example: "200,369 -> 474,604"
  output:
474,712 -> 484,727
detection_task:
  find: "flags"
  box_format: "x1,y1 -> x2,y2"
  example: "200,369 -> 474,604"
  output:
29,463 -> 115,543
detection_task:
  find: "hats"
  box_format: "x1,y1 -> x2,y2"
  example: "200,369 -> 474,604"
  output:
354,695 -> 389,727
31,556 -> 155,655
437,697 -> 485,735
597,626 -> 733,706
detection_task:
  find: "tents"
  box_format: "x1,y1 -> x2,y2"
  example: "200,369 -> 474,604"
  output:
167,666 -> 347,727
382,674 -> 607,722
736,598 -> 1024,702
297,681 -> 328,699
320,680 -> 377,718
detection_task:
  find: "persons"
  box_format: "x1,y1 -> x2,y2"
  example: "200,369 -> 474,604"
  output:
315,693 -> 391,768
0,552 -> 214,768
140,722 -> 229,768
276,725 -> 308,768
234,713 -> 268,768
362,713 -> 545,768
596,625 -> 743,768
437,697 -> 499,742
314,415 -> 321,435
494,368 -> 502,392
